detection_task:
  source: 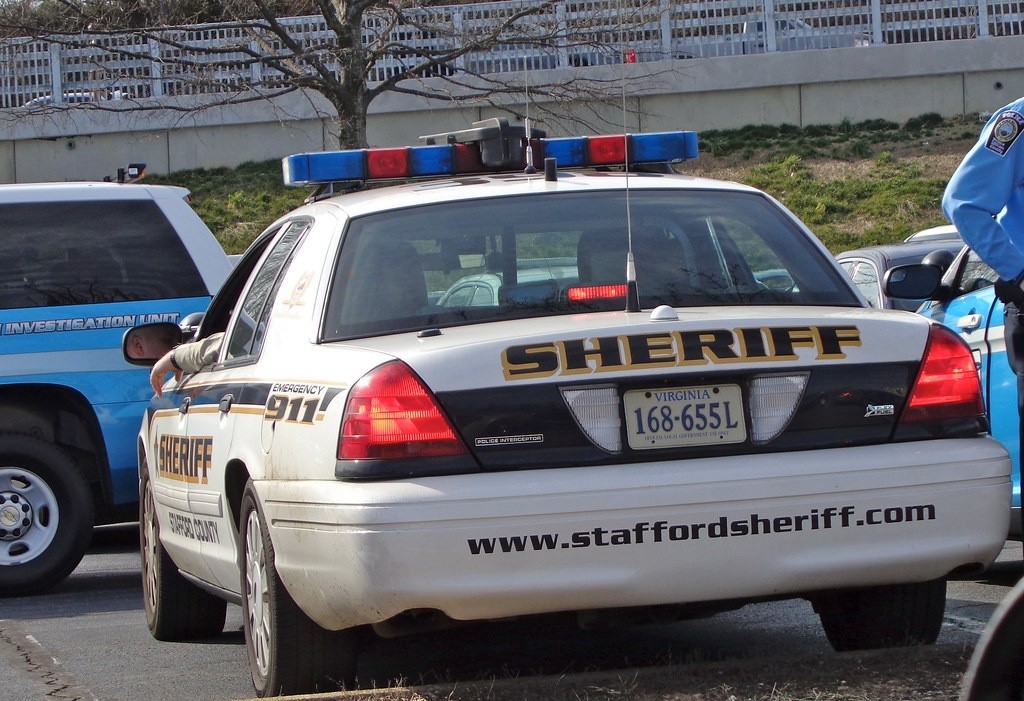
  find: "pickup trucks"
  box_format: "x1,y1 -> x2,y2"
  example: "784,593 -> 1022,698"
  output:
625,11 -> 888,65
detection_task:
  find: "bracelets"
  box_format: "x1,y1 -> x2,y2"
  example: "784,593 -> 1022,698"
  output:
170,346 -> 183,371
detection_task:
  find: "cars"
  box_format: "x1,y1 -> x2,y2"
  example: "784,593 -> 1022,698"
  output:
20,91 -> 136,109
122,50 -> 1015,699
433,256 -> 581,311
749,223 -> 1024,542
433,21 -> 638,74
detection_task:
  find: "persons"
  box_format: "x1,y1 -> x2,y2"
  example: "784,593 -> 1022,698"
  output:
149,332 -> 226,398
941,97 -> 1024,561
126,323 -> 179,358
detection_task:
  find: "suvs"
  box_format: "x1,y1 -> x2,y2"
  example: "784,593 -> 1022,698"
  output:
0,182 -> 265,600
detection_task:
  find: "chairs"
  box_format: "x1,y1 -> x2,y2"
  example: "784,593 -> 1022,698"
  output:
639,213 -> 756,296
577,228 -> 642,284
345,240 -> 428,324
